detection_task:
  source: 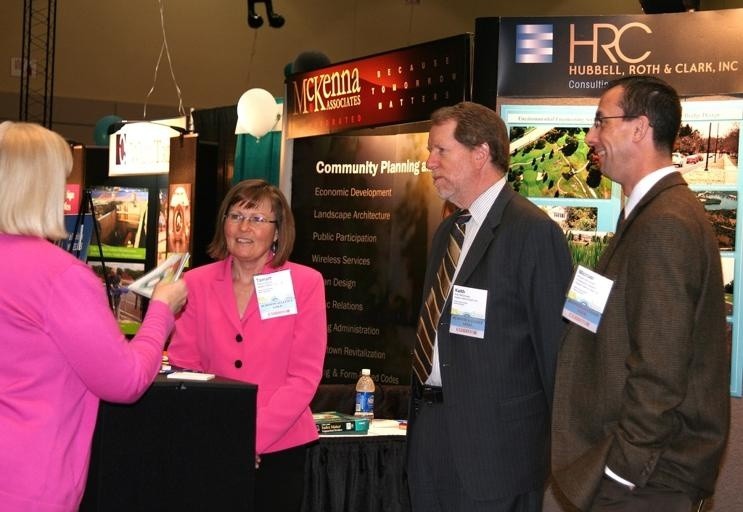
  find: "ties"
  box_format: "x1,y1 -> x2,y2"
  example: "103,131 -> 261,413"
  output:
410,208 -> 471,384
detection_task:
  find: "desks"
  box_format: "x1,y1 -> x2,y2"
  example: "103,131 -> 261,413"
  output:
254,413 -> 410,510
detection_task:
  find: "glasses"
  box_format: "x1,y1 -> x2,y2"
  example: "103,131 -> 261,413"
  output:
594,115 -> 636,126
224,212 -> 277,224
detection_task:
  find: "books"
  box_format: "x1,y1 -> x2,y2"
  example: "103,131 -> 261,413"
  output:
166,371 -> 216,382
303,408 -> 369,436
128,250 -> 191,302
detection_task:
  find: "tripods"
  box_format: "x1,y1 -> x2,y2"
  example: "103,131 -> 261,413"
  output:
67,190 -> 115,315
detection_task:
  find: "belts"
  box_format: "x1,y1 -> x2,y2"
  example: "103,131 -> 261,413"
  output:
410,386 -> 443,403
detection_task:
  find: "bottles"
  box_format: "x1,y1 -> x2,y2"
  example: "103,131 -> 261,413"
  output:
353,368 -> 375,421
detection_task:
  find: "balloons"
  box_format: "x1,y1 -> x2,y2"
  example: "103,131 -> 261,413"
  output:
234,86 -> 279,142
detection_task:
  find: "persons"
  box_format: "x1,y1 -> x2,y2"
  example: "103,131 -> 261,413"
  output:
552,78 -> 725,512
0,119 -> 189,509
408,100 -> 570,511
164,179 -> 327,511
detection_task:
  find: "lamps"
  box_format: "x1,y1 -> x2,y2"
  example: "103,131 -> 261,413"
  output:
92,114 -> 189,143
246,0 -> 284,29
